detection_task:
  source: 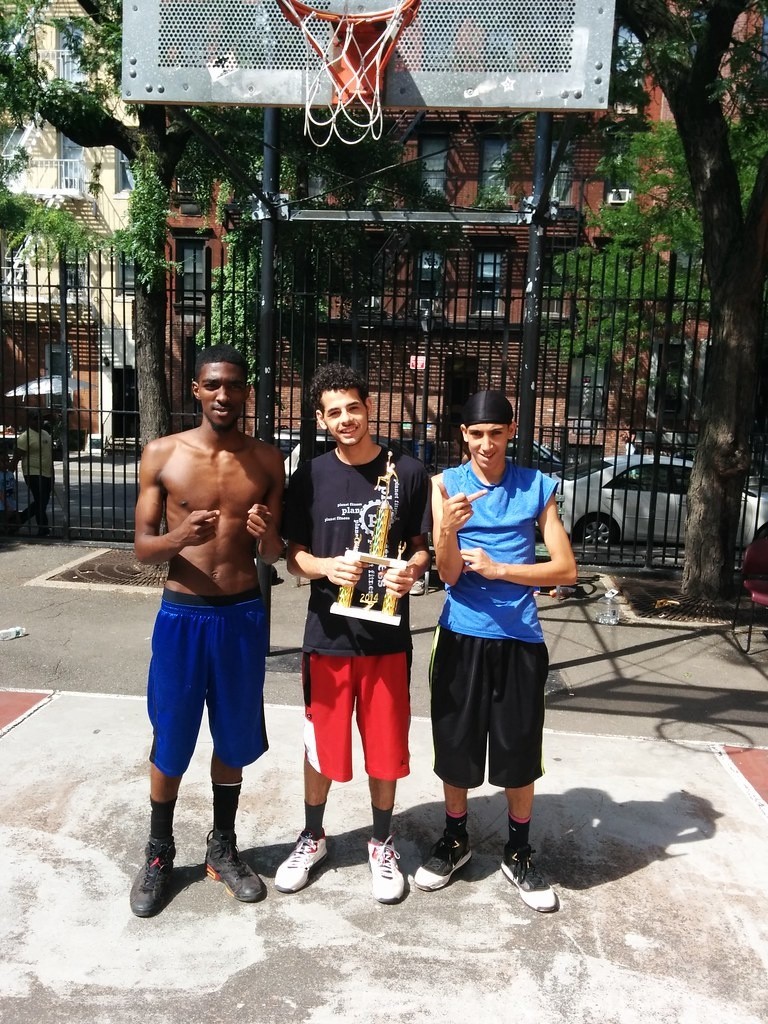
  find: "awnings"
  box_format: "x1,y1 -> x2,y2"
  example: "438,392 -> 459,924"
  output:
631,431 -> 699,449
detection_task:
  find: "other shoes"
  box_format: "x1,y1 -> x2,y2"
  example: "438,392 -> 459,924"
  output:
18,511 -> 24,528
38,527 -> 53,537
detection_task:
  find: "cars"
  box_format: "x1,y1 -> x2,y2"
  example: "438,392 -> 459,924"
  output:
505,435 -> 567,473
274,428 -> 434,491
536,454 -> 768,546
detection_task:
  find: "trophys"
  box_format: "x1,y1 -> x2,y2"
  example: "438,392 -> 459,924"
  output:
329,450 -> 408,626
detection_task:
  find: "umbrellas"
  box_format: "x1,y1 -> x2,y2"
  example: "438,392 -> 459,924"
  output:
3,374 -> 98,402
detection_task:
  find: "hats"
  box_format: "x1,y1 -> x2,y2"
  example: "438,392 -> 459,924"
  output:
462,390 -> 513,428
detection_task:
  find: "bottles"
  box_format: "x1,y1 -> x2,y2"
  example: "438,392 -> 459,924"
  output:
549,586 -> 578,597
594,589 -> 621,626
0,626 -> 26,641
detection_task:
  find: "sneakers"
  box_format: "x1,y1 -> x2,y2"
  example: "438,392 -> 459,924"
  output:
205,828 -> 263,902
415,828 -> 472,891
275,828 -> 327,893
130,835 -> 176,918
500,841 -> 559,912
367,835 -> 405,905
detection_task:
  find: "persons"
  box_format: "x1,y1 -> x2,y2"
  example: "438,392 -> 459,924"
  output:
129,345 -> 286,918
275,363 -> 434,904
0,450 -> 17,534
7,410 -> 54,536
415,391 -> 577,912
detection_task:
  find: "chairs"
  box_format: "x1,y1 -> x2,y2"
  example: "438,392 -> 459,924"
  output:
730,538 -> 768,654
